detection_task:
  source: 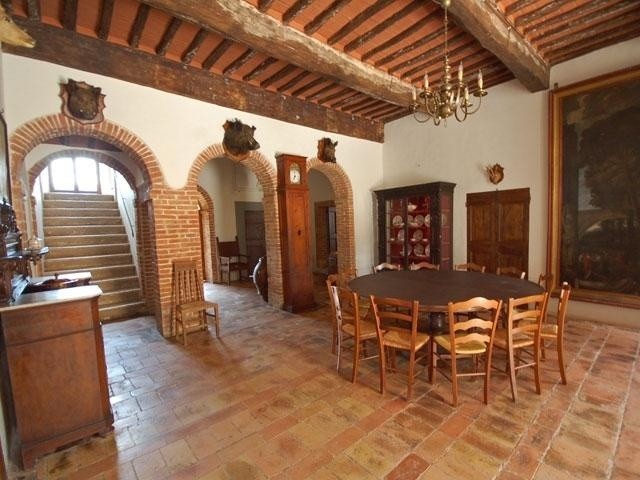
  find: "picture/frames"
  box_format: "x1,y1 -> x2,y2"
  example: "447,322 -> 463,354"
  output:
545,63 -> 640,311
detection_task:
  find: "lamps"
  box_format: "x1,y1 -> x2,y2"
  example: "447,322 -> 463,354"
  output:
409,0 -> 488,128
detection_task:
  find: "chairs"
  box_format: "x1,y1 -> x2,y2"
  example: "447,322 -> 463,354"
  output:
173,260 -> 220,350
326,261 -> 572,409
216,236 -> 250,286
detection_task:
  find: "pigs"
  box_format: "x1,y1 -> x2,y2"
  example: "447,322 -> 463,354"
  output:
67,79 -> 101,120
224,118 -> 260,153
323,138 -> 338,163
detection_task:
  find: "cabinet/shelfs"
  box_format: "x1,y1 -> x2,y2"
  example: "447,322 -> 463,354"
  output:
372,181 -> 457,272
